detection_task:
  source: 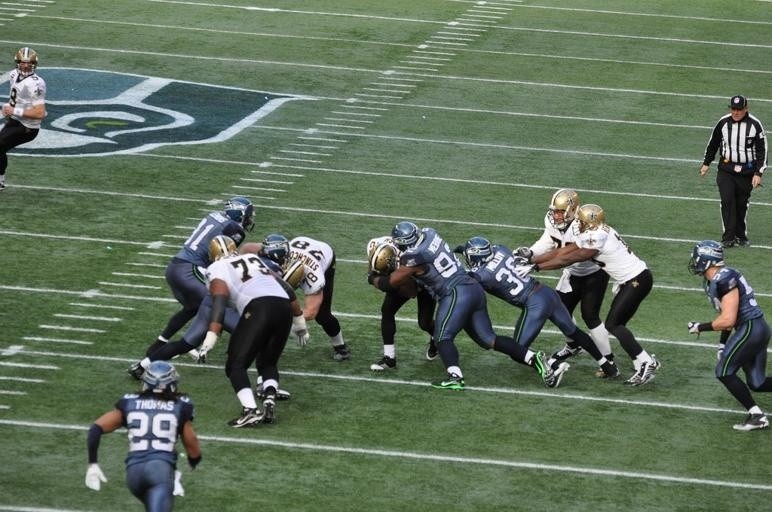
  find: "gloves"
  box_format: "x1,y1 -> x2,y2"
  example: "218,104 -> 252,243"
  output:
716,344 -> 725,360
196,332 -> 218,363
84,464 -> 108,492
687,321 -> 700,340
294,311 -> 311,346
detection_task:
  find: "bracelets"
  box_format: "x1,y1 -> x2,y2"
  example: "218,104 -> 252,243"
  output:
14,107 -> 24,118
719,329 -> 732,343
697,321 -> 714,332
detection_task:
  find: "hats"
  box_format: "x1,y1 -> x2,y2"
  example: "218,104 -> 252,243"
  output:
727,95 -> 747,111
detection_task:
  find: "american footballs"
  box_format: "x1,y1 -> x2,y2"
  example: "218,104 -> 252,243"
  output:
400,277 -> 418,298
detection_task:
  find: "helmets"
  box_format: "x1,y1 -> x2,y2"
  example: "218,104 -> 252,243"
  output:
207,197 -> 304,292
687,240 -> 725,278
367,189 -> 605,277
13,47 -> 39,78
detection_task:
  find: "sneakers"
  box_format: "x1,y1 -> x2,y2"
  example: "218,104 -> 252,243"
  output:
733,413 -> 769,431
735,236 -> 750,248
721,239 -> 734,248
127,343 -> 291,428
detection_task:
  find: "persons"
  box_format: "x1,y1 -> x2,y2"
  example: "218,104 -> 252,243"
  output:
453,237 -> 617,380
368,220 -> 555,389
513,204 -> 661,386
687,240 -> 772,430
85,360 -> 202,512
199,235 -> 309,426
512,188 -> 619,377
0,47 -> 46,191
700,94 -> 769,247
367,235 -> 439,370
146,198 -> 256,356
129,234 -> 352,400
